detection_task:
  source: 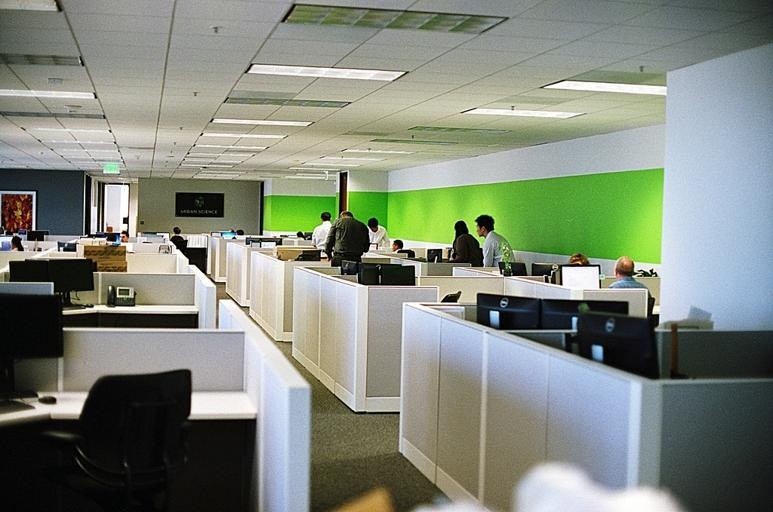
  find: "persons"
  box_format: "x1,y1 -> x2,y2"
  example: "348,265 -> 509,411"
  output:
475,215 -> 515,267
232,230 -> 244,239
366,217 -> 391,248
448,221 -> 483,267
11,237 -> 24,251
120,231 -> 129,242
170,227 -> 184,243
325,210 -> 370,267
392,240 -> 403,253
568,253 -> 589,265
312,211 -> 333,260
297,231 -> 307,240
608,256 -> 652,297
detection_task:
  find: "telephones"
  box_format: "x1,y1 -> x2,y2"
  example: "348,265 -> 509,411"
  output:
107,284 -> 137,306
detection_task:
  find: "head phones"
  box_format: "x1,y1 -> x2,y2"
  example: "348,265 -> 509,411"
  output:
376,264 -> 383,285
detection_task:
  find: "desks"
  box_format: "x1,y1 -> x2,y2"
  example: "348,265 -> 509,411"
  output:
64,303 -> 198,314
0,391 -> 257,427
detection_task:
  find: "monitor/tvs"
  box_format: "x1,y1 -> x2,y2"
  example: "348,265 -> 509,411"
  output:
358,262 -> 401,284
57,241 -> 79,252
532,263 -> 553,276
261,238 -> 282,245
48,259 -> 94,307
477,293 -> 540,329
427,249 -> 442,262
361,266 -> 415,286
0,236 -> 13,251
541,298 -> 628,330
560,264 -> 601,290
498,262 -> 527,276
89,234 -> 105,238
0,282 -> 54,295
221,232 -> 237,237
10,261 -> 48,282
0,293 -> 64,399
369,243 -> 378,250
156,232 -> 169,240
342,259 -> 357,275
211,232 -> 221,236
105,233 -> 120,242
26,230 -> 49,241
245,238 -> 261,248
578,312 -> 660,380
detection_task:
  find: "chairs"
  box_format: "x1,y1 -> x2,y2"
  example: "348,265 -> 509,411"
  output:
441,292 -> 461,302
44,369 -> 192,511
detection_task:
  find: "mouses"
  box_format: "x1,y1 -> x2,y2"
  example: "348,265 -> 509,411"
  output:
39,396 -> 56,404
84,304 -> 93,307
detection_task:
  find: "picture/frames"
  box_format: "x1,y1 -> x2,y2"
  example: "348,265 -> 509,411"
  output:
0,191 -> 36,237
